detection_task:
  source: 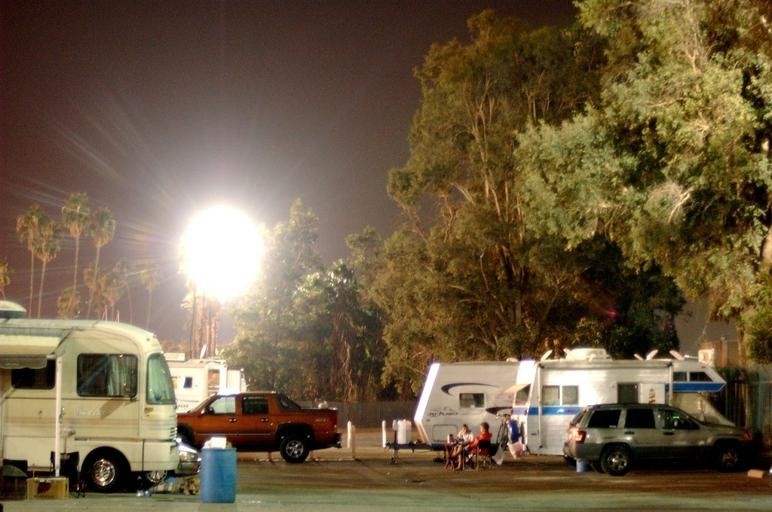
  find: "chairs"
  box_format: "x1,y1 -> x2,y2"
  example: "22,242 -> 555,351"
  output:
466,439 -> 492,470
49,451 -> 87,497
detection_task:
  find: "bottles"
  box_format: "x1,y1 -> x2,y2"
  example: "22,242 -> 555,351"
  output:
137,491 -> 152,496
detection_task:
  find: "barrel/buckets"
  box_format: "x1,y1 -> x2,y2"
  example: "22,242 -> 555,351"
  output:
200,448 -> 237,503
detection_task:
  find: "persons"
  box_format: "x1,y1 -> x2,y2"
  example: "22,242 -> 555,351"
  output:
501,412 -> 525,460
455,422 -> 476,470
448,421 -> 492,470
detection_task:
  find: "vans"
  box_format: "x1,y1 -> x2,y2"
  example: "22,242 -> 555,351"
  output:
0,299 -> 183,493
503,345 -> 736,459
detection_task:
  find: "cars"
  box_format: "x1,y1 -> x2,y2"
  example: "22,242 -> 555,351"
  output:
178,391 -> 342,463
561,402 -> 750,477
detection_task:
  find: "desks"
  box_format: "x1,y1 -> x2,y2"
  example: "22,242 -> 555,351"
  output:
442,442 -> 478,472
